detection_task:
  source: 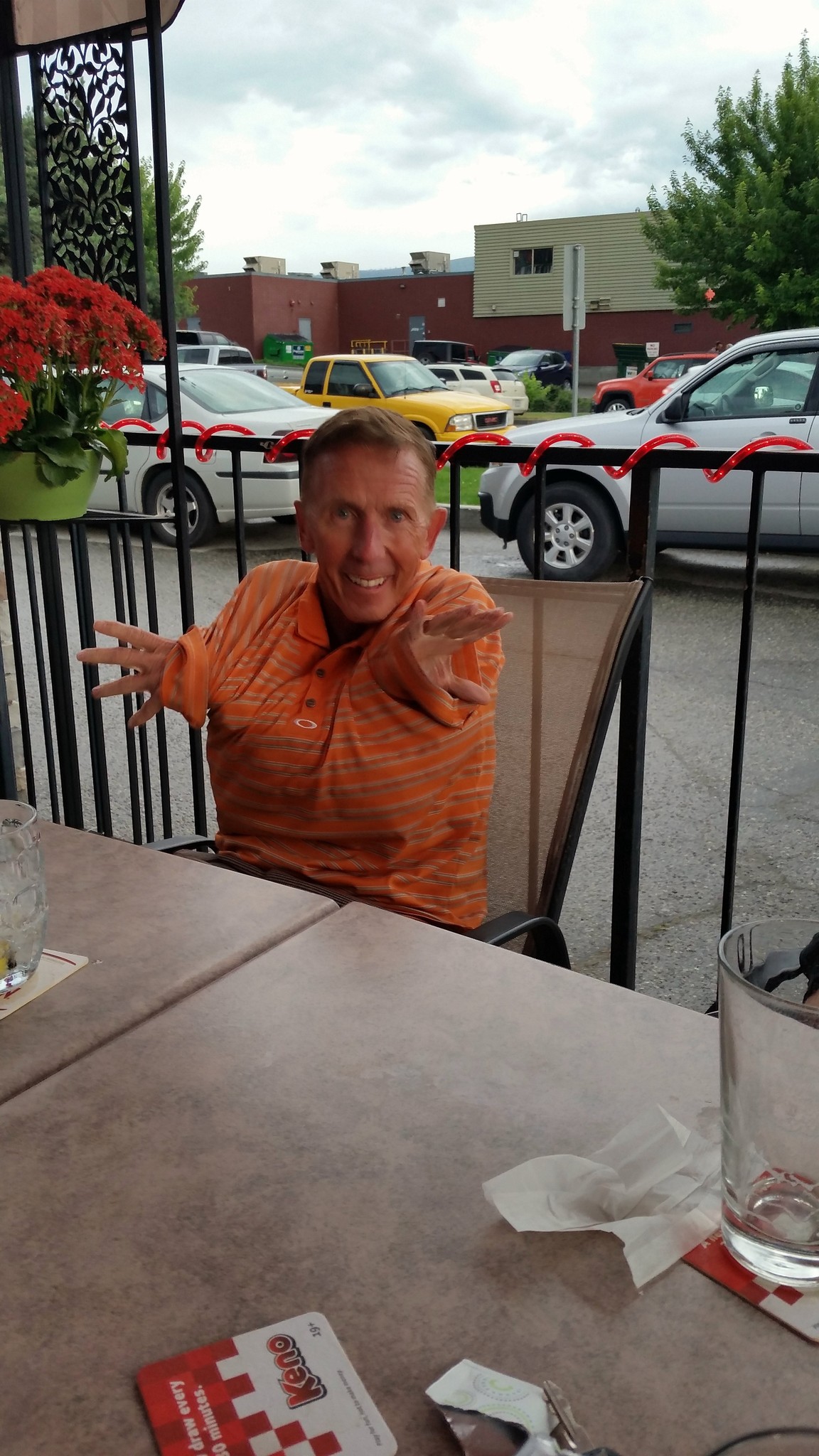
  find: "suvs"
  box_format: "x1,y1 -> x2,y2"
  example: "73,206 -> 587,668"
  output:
591,351 -> 718,414
663,359 -> 815,415
476,324 -> 819,582
411,340 -> 481,365
425,362 -> 529,417
175,328 -> 248,346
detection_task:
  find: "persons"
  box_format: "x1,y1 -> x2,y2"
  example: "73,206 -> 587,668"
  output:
78,407 -> 514,940
708,341 -> 733,355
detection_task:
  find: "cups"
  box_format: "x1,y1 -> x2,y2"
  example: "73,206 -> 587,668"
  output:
0,800 -> 43,993
717,918 -> 819,1286
707,1427 -> 819,1456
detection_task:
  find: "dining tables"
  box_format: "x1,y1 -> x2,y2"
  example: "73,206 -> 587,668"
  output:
0,900 -> 819,1456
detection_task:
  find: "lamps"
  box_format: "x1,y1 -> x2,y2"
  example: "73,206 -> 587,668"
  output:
492,304 -> 497,311
599,304 -> 612,310
600,297 -> 611,302
399,284 -> 406,288
590,300 -> 599,309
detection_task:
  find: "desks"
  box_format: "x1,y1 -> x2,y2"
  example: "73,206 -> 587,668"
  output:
0,811 -> 341,1104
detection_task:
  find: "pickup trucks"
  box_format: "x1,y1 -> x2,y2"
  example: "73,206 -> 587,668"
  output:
275,352 -> 515,444
162,344 -> 268,380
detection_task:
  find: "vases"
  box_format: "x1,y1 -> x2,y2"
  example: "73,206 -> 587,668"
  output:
0,449 -> 104,521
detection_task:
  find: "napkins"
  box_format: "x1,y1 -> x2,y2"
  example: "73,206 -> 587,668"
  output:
480,1106 -> 770,1288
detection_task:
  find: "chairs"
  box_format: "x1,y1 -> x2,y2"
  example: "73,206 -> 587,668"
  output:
140,576 -> 654,971
102,399 -> 124,423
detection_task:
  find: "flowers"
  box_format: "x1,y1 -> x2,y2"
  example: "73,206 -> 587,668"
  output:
0,266 -> 168,487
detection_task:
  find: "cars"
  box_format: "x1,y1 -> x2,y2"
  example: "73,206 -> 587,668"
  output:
492,349 -> 573,392
0,365 -> 344,542
